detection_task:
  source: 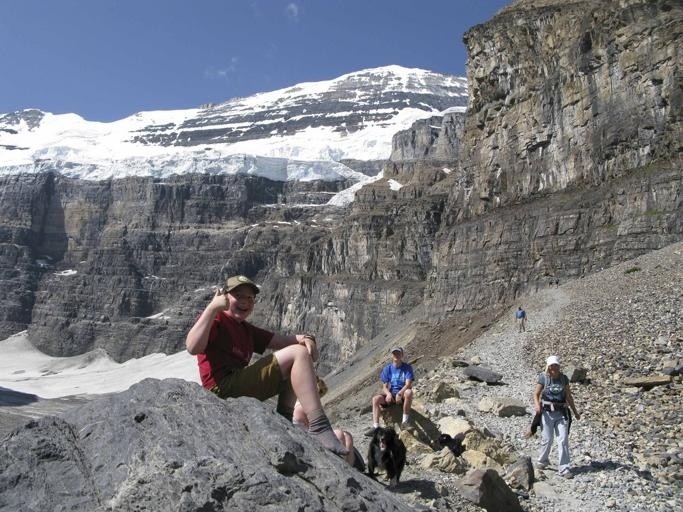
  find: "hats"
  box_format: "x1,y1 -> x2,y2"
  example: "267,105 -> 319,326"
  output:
223,274 -> 260,295
392,345 -> 403,353
547,356 -> 560,366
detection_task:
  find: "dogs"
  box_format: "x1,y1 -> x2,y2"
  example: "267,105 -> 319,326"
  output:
367,425 -> 407,488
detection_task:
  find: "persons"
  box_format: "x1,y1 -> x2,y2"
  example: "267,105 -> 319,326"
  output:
364,344 -> 415,437
290,373 -> 355,466
183,275 -> 351,457
515,306 -> 527,333
533,355 -> 582,479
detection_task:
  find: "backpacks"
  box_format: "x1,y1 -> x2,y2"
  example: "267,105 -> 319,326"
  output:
540,374 -> 567,412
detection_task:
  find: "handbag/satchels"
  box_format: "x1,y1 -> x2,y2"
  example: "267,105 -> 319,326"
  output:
430,434 -> 466,457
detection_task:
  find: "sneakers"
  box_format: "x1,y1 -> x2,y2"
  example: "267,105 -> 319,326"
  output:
562,471 -> 574,479
536,464 -> 545,470
364,426 -> 377,436
400,422 -> 415,431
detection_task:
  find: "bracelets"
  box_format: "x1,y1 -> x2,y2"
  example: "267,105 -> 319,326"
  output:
301,335 -> 316,345
397,393 -> 402,396
